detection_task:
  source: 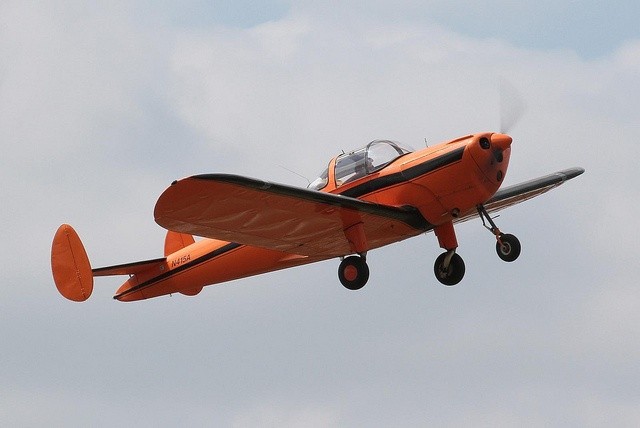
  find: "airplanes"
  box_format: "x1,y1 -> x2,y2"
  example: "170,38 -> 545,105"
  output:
51,133 -> 585,302
